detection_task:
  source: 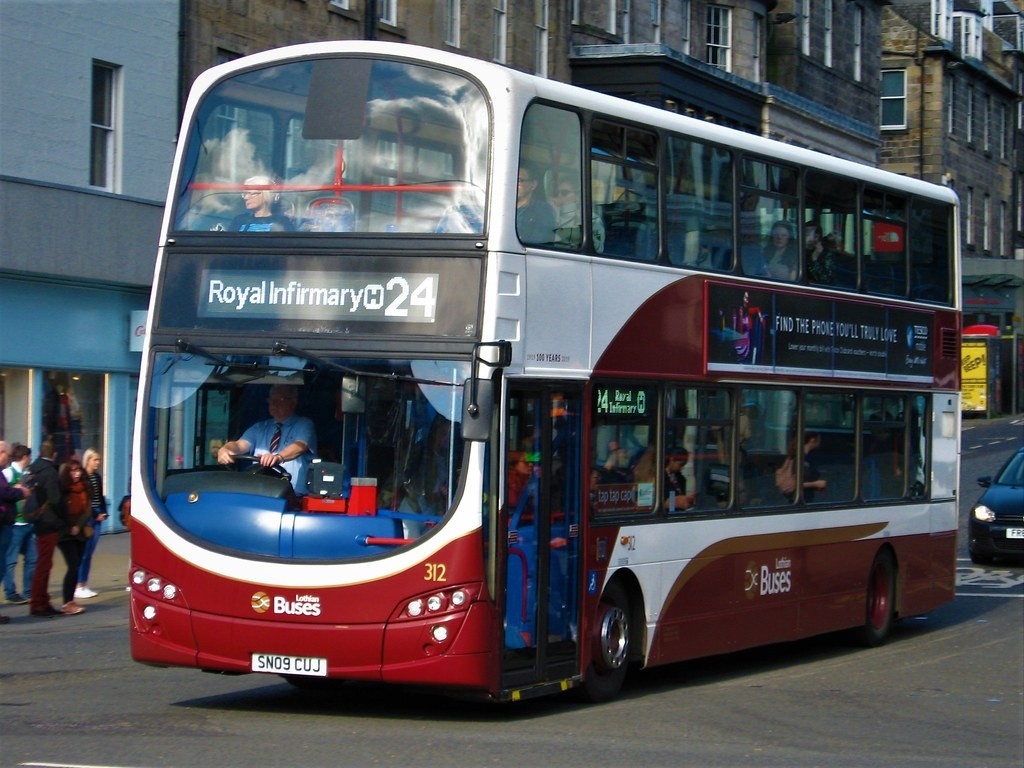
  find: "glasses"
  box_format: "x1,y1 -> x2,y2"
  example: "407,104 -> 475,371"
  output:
70,467 -> 81,472
242,192 -> 260,199
551,189 -> 578,198
517,177 -> 531,182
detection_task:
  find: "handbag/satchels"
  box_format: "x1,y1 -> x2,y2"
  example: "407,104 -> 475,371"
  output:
23,491 -> 45,522
776,459 -> 795,493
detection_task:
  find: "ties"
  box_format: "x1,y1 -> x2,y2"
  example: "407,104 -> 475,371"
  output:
270,423 -> 282,454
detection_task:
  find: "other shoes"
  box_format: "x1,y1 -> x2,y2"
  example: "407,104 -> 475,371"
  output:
61,601 -> 85,615
31,607 -> 65,618
0,615 -> 9,623
4,594 -> 28,605
73,586 -> 98,598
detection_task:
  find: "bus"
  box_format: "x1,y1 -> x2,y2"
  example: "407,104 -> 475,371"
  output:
126,38 -> 963,706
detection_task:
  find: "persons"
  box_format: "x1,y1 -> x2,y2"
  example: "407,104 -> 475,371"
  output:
208,176 -> 294,233
507,379 -> 918,538
494,158 -> 847,293
388,412 -> 465,538
216,381 -> 318,505
0,439 -> 109,624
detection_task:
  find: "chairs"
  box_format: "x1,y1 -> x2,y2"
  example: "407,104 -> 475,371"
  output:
710,460 -> 879,506
284,197 -> 763,273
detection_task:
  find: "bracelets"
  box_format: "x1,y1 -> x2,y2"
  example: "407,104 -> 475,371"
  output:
275,453 -> 286,464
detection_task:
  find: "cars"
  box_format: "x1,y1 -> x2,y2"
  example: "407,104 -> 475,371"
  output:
968,446 -> 1024,566
